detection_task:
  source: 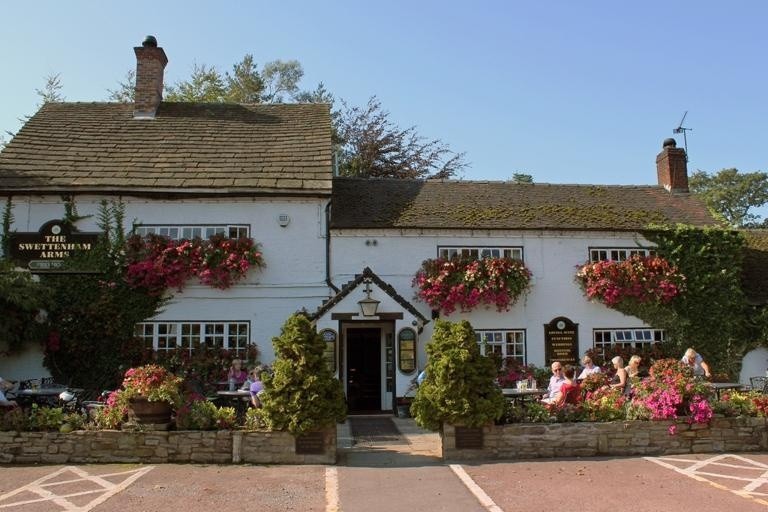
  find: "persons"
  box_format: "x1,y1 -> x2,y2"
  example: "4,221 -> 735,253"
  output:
681,348 -> 712,378
576,354 -> 602,379
738,340 -> 768,393
0,376 -> 19,410
592,354 -> 627,396
240,369 -> 255,402
250,363 -> 270,409
542,360 -> 567,398
537,364 -> 581,411
229,358 -> 247,383
624,354 -> 642,395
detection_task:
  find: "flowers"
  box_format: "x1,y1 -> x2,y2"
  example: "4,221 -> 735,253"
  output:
573,255 -> 688,311
114,231 -> 268,295
543,356 -> 767,437
91,344 -> 259,430
410,254 -> 535,319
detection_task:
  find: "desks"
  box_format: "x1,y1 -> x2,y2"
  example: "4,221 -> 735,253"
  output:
501,388 -> 547,407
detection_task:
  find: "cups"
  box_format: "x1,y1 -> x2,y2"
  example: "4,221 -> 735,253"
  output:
532,380 -> 536,389
522,380 -> 528,392
516,381 -> 521,391
